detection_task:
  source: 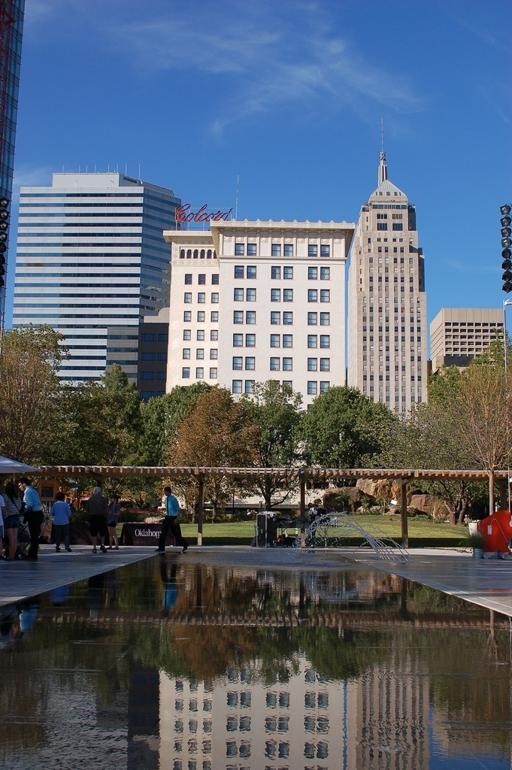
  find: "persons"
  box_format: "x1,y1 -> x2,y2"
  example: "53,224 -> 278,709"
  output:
296,527 -> 307,549
4,482 -> 23,562
86,575 -> 103,622
47,586 -> 71,608
308,505 -> 319,520
155,486 -> 189,553
18,478 -> 45,560
157,559 -> 180,618
272,528 -> 288,546
87,486 -> 108,554
50,491 -> 73,553
0,494 -> 6,562
104,573 -> 118,612
14,596 -> 41,640
106,494 -> 122,551
0,604 -> 18,637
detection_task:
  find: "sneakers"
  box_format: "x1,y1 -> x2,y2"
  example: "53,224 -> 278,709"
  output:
182,544 -> 189,551
65,547 -> 72,552
155,548 -> 164,551
56,549 -> 60,552
93,546 -> 119,554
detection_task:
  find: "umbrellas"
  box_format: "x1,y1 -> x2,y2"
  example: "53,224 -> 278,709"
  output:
1,454 -> 40,475
